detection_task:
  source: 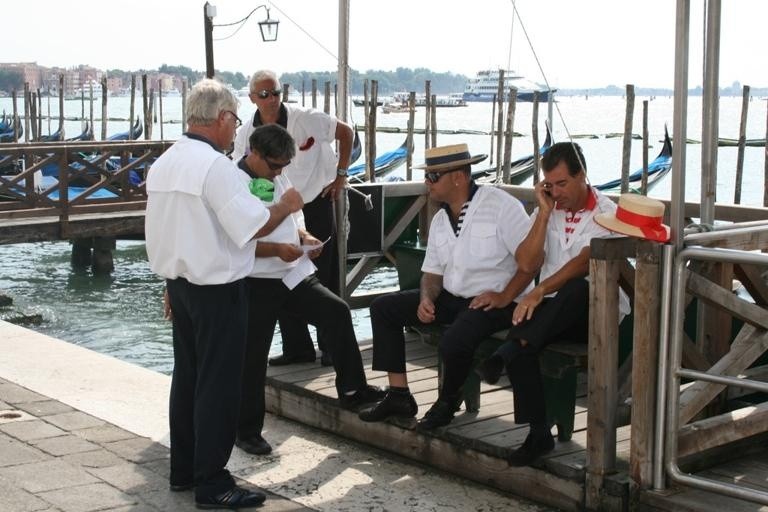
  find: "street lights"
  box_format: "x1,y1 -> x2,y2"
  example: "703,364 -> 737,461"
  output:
201,1 -> 280,81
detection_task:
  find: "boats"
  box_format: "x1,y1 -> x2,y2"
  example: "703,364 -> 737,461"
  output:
345,117 -> 414,184
351,88 -> 468,113
0,108 -> 169,201
347,116 -> 673,260
464,66 -> 557,105
349,124 -> 363,167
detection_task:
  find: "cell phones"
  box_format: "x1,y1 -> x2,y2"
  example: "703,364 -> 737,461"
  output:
544,185 -> 551,197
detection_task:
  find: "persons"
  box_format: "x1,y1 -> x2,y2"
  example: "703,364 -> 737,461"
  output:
232,67 -> 360,368
502,140 -> 637,466
356,140 -> 546,432
139,77 -> 307,511
232,122 -> 386,457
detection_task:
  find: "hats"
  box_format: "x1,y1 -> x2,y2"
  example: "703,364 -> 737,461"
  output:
593,194 -> 671,242
409,144 -> 488,170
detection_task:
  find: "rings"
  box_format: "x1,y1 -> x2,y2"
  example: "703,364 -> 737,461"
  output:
521,304 -> 528,308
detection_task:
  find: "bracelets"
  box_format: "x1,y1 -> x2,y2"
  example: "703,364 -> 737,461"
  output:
300,232 -> 312,246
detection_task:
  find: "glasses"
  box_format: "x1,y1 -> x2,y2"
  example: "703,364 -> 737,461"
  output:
228,109 -> 242,128
265,158 -> 290,169
425,171 -> 455,183
252,89 -> 282,98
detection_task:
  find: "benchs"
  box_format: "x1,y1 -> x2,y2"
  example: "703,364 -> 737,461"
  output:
409,315 -> 589,442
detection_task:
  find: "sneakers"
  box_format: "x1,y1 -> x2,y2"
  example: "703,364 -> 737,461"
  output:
235,432 -> 272,454
339,383 -> 388,408
321,351 -> 333,366
171,478 -> 265,507
475,351 -> 504,384
359,389 -> 417,422
269,349 -> 317,365
512,429 -> 555,466
420,390 -> 466,429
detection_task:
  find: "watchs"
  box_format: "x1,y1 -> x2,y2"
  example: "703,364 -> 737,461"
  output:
337,169 -> 349,177
